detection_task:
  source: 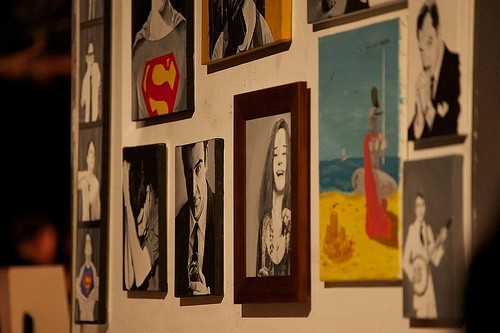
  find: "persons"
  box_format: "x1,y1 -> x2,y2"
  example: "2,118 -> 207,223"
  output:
402,195 -> 448,319
255,119 -> 293,278
174,141 -> 220,298
406,4 -> 460,143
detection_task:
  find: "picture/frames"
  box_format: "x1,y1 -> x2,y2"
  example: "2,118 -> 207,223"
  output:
201,0 -> 293,66
64,0 -> 114,325
233,79 -> 314,307
